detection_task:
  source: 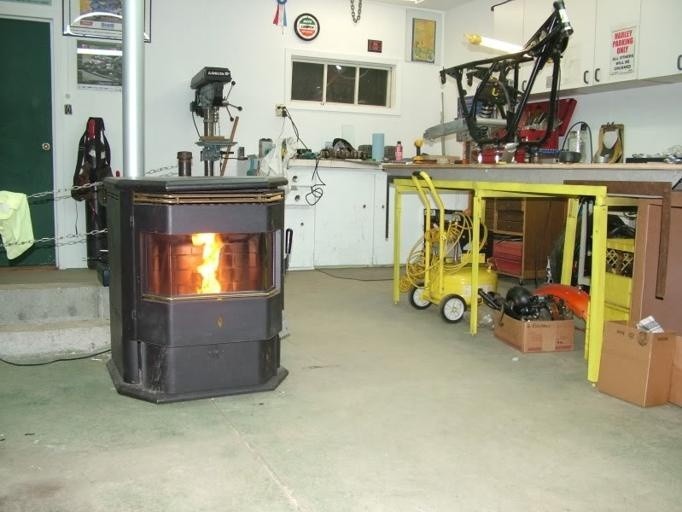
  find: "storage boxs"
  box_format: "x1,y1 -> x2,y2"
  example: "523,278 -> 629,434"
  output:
493,309 -> 577,354
597,318 -> 682,409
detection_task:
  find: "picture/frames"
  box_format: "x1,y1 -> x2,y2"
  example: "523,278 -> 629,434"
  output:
61,0 -> 154,91
402,8 -> 446,70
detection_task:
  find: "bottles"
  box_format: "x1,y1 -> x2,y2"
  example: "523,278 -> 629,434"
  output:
568,123 -> 588,163
371,132 -> 385,161
395,141 -> 403,161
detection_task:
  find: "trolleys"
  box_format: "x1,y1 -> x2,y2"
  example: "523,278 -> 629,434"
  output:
409,169 -> 500,323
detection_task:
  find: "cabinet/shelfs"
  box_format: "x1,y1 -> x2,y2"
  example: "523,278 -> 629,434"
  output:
480,198 -> 564,285
283,167 -> 470,271
489,1 -> 681,100
